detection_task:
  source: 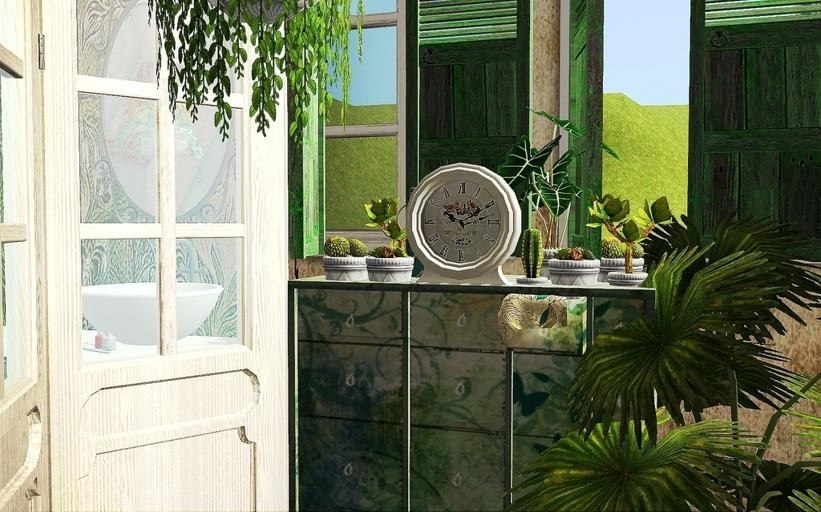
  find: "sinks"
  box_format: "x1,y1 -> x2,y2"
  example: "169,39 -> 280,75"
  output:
82,283 -> 223,345
